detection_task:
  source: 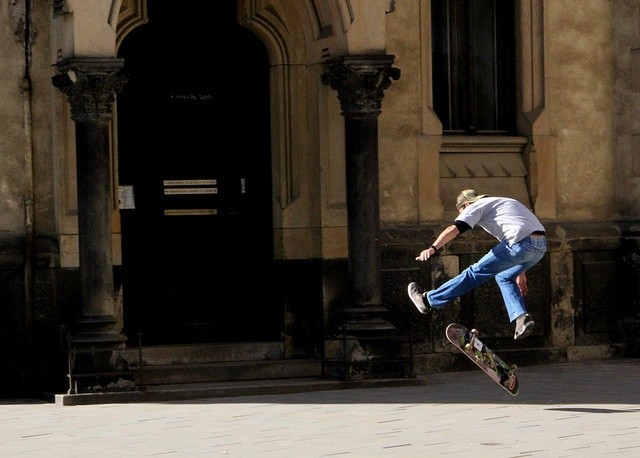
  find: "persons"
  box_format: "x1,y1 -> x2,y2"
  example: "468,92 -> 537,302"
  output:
407,189 -> 547,340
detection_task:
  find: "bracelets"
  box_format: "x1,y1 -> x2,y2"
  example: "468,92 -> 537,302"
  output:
432,245 -> 437,252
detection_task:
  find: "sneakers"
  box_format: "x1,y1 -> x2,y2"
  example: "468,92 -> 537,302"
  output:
514,313 -> 535,340
408,282 -> 432,314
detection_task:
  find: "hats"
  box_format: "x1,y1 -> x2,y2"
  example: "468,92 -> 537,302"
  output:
456,189 -> 490,210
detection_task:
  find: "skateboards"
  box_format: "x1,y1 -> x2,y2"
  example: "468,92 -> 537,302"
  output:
445,323 -> 521,396
